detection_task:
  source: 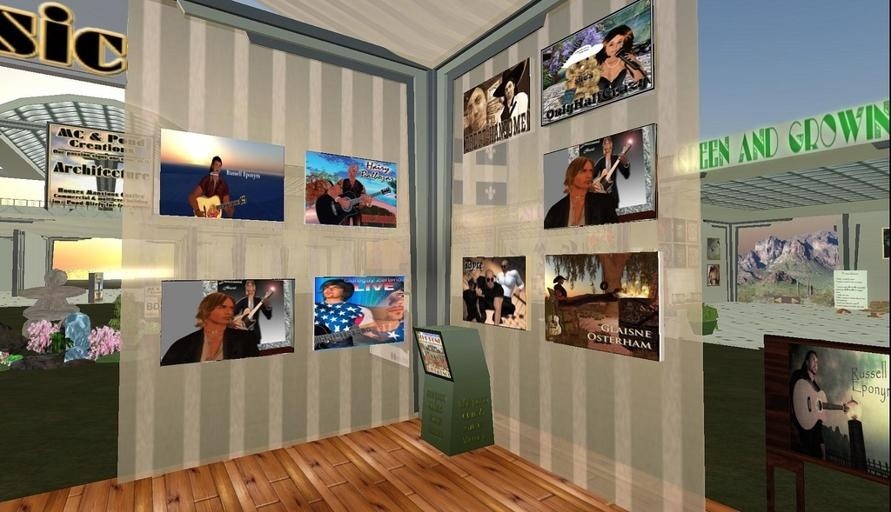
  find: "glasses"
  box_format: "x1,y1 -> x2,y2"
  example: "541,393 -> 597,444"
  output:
487,278 -> 495,283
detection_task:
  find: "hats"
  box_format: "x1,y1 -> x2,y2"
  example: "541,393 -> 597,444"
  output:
561,44 -> 604,71
493,58 -> 528,98
553,276 -> 568,283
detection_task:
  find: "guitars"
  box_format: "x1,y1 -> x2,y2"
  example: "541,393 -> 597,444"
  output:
794,378 -> 851,429
547,296 -> 563,337
191,194 -> 247,218
233,290 -> 274,330
316,187 -> 392,224
590,142 -> 630,192
